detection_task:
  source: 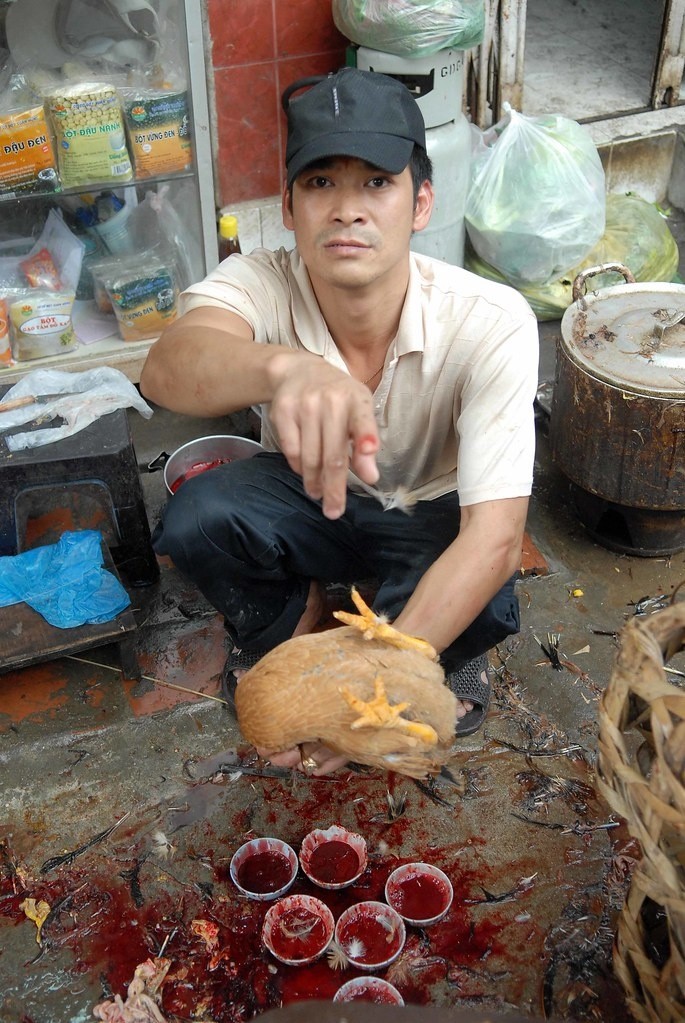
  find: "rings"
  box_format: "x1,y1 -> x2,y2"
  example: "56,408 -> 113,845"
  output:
301,756 -> 319,777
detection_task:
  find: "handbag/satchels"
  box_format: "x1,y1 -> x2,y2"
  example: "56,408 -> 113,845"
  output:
332,0 -> 484,58
466,101 -> 680,323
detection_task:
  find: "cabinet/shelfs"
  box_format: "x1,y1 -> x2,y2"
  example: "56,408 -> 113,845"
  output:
1,0 -> 220,388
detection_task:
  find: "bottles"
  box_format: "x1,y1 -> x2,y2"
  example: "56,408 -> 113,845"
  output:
218,215 -> 242,263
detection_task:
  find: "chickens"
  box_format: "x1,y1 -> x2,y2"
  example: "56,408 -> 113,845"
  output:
235,586 -> 458,780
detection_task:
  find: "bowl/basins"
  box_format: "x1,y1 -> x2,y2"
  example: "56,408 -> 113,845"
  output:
299,825 -> 368,889
335,901 -> 406,971
229,837 -> 298,901
332,976 -> 405,1007
262,894 -> 335,966
385,862 -> 454,926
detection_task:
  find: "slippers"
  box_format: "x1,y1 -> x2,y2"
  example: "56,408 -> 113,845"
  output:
444,649 -> 491,737
222,646 -> 268,720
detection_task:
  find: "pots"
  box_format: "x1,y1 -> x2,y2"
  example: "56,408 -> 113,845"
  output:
149,434 -> 264,497
552,263 -> 685,510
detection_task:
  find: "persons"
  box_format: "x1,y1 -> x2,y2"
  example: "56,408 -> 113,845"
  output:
140,68 -> 539,777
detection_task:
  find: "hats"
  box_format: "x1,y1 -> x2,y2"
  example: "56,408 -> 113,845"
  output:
285,66 -> 426,189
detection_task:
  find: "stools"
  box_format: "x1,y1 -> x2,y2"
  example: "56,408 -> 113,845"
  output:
0,383 -> 162,588
0,538 -> 140,681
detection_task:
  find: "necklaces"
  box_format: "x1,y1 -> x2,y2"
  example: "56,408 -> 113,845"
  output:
361,364 -> 384,384
